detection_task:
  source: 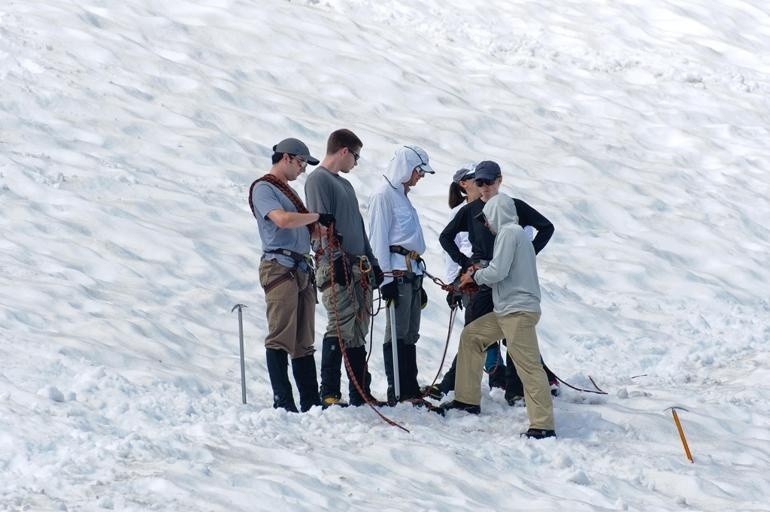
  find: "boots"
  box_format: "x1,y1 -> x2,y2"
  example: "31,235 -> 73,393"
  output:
264,335 -> 432,414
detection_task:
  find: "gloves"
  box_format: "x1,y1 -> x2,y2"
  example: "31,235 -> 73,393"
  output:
321,213 -> 428,306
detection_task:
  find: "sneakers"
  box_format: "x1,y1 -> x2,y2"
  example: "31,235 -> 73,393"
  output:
423,385 -> 561,440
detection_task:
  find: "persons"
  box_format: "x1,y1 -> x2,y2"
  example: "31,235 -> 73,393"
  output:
367,142 -> 438,400
302,127 -> 385,408
247,138 -> 331,412
441,163 -> 564,396
430,161 -> 555,406
432,193 -> 559,441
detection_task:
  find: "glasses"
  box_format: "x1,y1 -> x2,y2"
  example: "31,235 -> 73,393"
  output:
344,146 -> 361,160
415,166 -> 425,176
290,155 -> 307,168
476,176 -> 501,187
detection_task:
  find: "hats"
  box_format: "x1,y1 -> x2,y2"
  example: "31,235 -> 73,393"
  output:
420,162 -> 435,174
276,139 -> 320,166
473,212 -> 485,224
475,161 -> 501,181
453,167 -> 475,183
